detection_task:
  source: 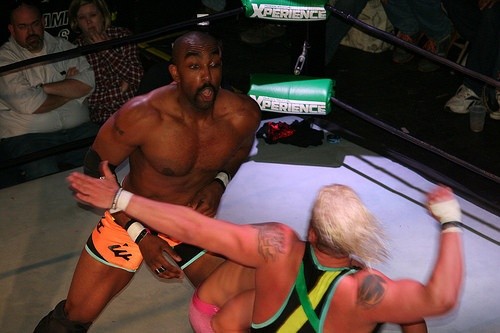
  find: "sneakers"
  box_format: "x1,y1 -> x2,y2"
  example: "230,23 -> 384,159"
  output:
418,39 -> 448,72
490,84 -> 500,120
445,84 -> 487,113
392,31 -> 423,64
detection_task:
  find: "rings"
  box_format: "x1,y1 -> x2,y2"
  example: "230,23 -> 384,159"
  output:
155,266 -> 165,274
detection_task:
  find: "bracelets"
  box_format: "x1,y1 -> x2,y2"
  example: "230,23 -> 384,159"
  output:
112,187 -> 134,211
215,170 -> 230,189
125,219 -> 147,244
442,221 -> 461,230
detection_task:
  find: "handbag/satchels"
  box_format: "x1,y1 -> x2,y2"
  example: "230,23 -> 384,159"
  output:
256,120 -> 325,149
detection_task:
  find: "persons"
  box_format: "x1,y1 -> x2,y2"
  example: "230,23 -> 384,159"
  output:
68,161 -> 463,333
380,0 -> 500,113
188,259 -> 257,333
0,0 -> 95,183
34,32 -> 262,333
67,0 -> 145,126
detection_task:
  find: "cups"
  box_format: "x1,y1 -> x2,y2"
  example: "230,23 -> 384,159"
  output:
469,105 -> 487,132
